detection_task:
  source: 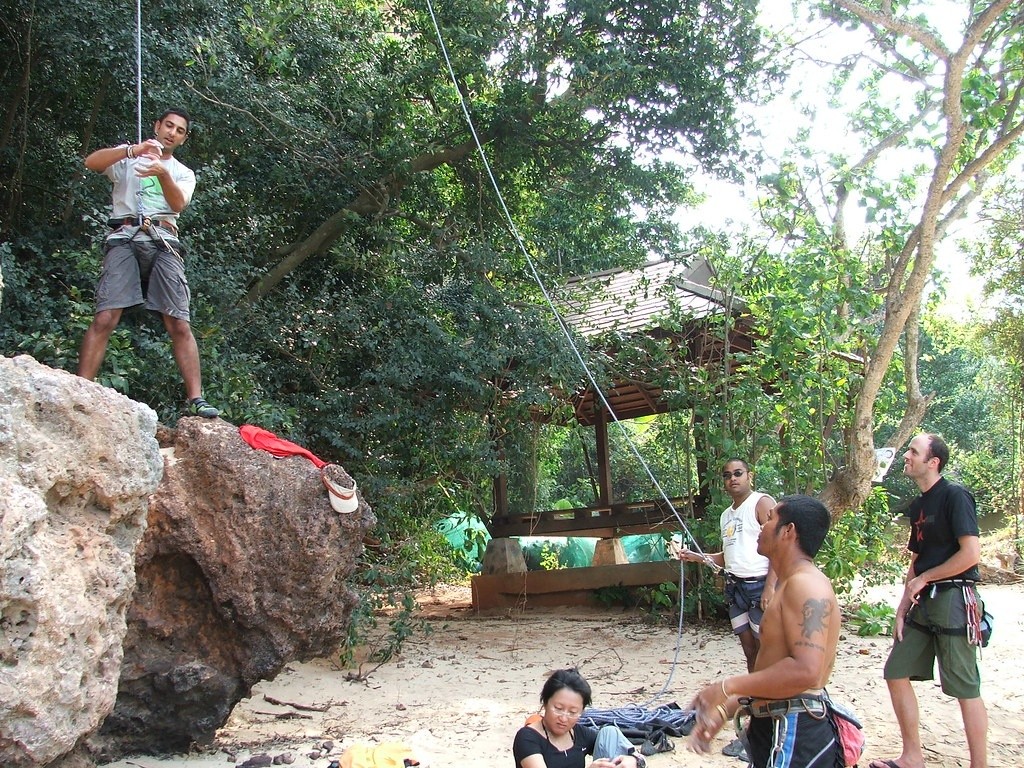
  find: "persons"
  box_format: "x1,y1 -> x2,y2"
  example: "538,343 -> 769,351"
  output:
79,106 -> 218,418
685,494 -> 845,768
678,458 -> 777,673
513,667 -> 648,768
869,433 -> 989,768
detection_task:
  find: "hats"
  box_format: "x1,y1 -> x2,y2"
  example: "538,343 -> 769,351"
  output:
321,464 -> 359,514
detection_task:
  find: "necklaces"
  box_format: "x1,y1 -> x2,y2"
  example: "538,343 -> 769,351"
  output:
734,495 -> 749,508
548,730 -> 567,758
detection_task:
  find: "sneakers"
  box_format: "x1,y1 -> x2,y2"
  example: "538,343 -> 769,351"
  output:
188,397 -> 218,418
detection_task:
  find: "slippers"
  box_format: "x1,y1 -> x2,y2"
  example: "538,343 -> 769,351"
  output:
869,759 -> 900,768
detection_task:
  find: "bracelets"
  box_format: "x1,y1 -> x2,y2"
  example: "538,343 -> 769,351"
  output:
632,755 -> 646,768
127,145 -> 137,160
716,703 -> 727,722
721,680 -> 729,699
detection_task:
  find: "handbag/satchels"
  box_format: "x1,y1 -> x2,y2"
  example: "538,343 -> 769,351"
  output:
828,698 -> 865,767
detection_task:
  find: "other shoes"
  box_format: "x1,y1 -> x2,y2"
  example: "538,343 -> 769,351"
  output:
641,730 -> 674,756
723,739 -> 744,755
738,748 -> 750,762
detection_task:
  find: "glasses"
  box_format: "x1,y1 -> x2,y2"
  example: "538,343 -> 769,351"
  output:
546,702 -> 582,719
722,469 -> 746,480
